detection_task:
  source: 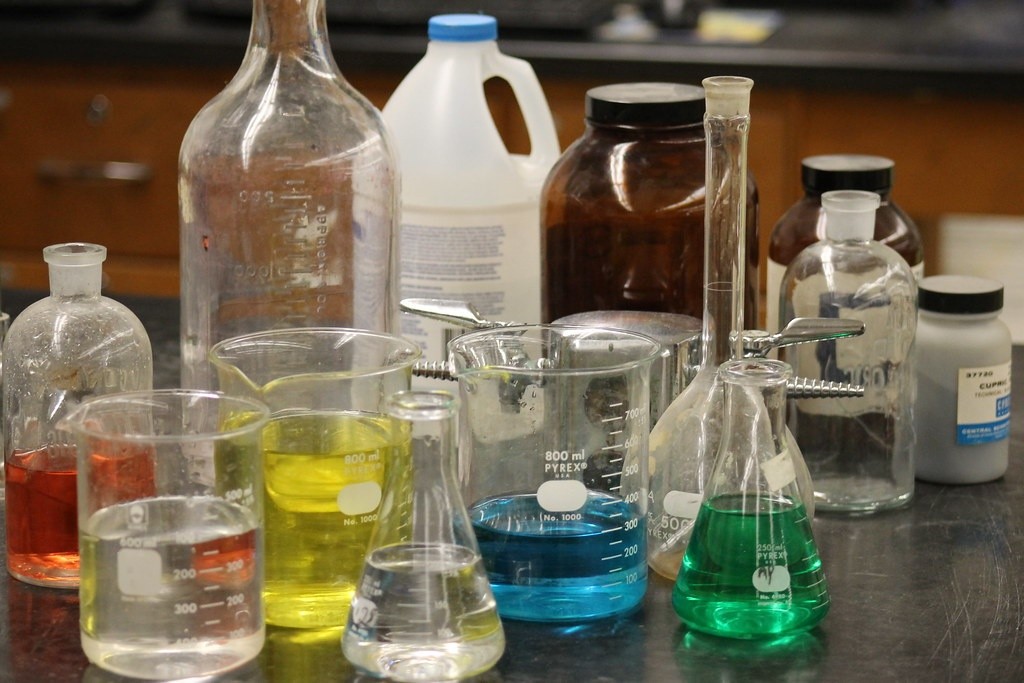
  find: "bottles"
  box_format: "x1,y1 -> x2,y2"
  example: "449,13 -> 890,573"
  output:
916,275 -> 1011,483
181,0 -> 396,488
779,190 -> 917,518
541,81 -> 759,494
768,156 -> 923,362
2,242 -> 153,587
352,14 -> 561,443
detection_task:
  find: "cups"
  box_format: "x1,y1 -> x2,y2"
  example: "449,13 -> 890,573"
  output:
209,328 -> 424,628
55,389 -> 270,681
449,326 -> 669,625
